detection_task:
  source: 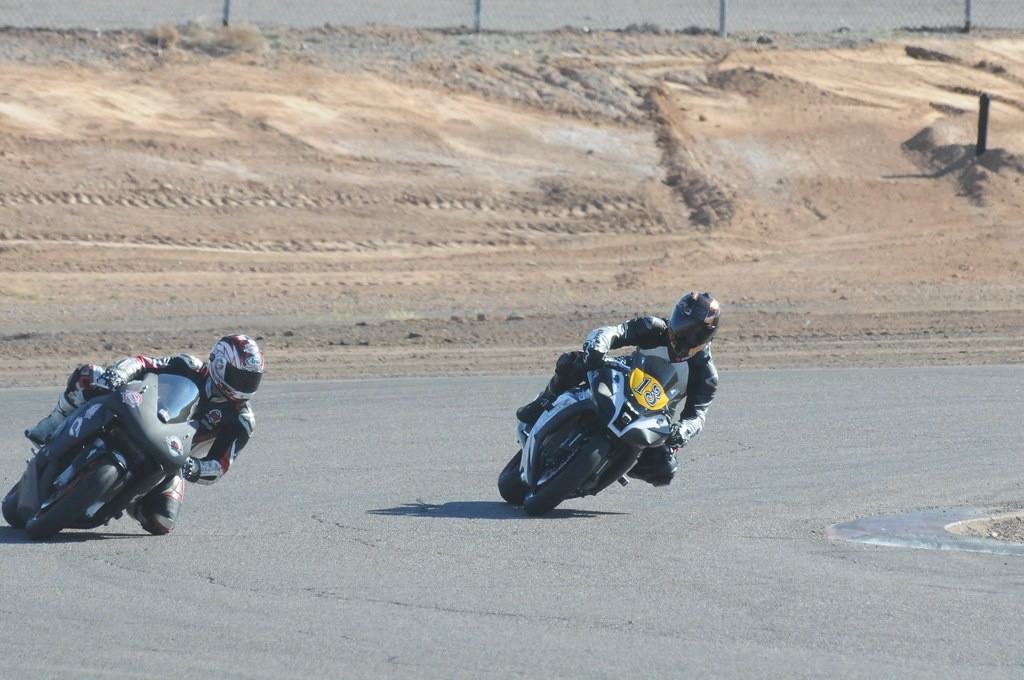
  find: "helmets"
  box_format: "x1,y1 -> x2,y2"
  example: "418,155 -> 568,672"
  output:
208,334 -> 264,403
666,291 -> 720,364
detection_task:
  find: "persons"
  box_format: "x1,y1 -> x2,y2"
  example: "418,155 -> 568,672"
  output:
25,334 -> 264,535
516,291 -> 722,485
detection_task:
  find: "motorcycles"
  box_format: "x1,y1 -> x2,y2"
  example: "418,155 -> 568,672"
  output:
498,325 -> 680,518
2,367 -> 202,540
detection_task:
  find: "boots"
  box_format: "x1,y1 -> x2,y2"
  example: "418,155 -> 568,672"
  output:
25,393 -> 79,444
516,373 -> 572,421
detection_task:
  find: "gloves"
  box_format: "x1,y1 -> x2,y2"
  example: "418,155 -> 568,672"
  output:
97,370 -> 124,392
179,457 -> 200,481
583,343 -> 605,369
668,422 -> 689,448
